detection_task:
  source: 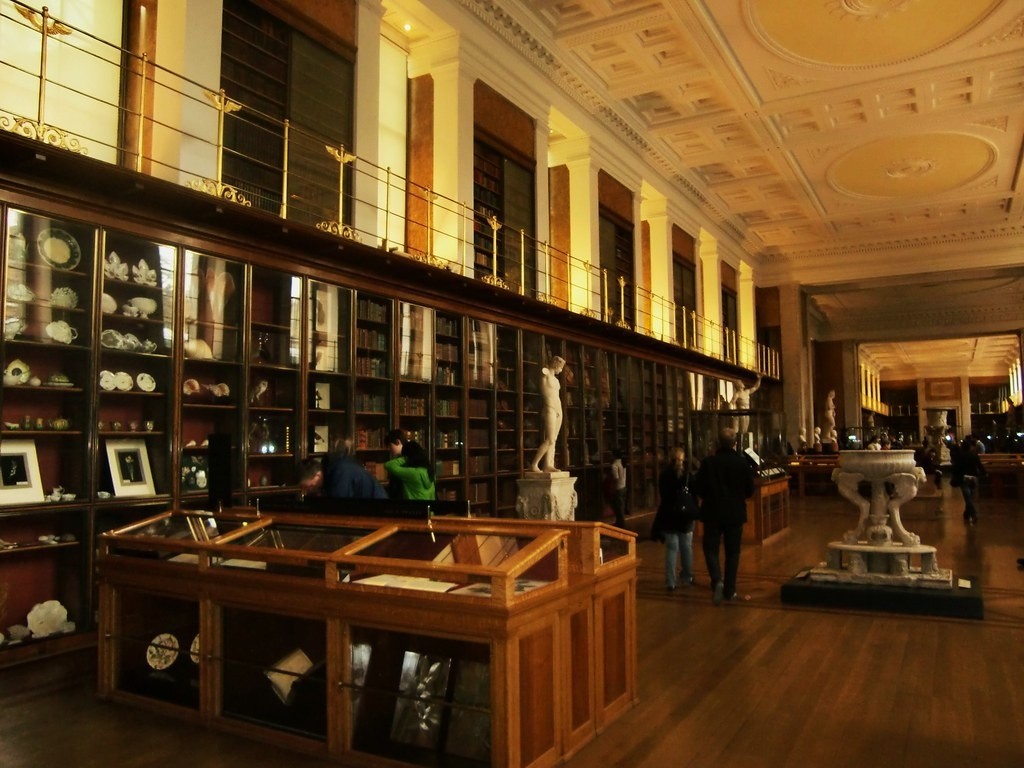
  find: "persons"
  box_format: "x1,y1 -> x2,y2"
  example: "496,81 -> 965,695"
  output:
838,427 -> 853,450
831,430 -> 837,443
659,447 -> 699,590
693,427 -> 755,601
814,427 -> 821,443
607,448 -> 626,528
920,449 -> 944,489
950,434 -> 989,524
798,428 -> 807,446
729,373 -> 763,431
972,433 -> 985,454
824,389 -> 836,439
530,356 -> 566,472
288,448 -> 389,520
383,430 -> 435,500
865,434 -> 904,500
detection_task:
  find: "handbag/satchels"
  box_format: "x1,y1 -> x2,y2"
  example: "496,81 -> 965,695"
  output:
665,487 -> 692,516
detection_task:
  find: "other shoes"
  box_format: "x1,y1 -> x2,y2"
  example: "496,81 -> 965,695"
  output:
669,585 -> 676,591
1017,558 -> 1024,566
712,582 -> 724,606
680,572 -> 694,585
725,593 -> 737,601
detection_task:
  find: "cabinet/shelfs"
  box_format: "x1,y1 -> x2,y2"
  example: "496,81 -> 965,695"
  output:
0,185 -> 691,699
94,510 -> 639,768
798,454 -> 839,495
978,452 -> 1024,496
697,469 -> 793,546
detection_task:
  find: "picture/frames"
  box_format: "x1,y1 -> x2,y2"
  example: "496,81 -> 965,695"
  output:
105,437 -> 156,497
0,439 -> 46,505
